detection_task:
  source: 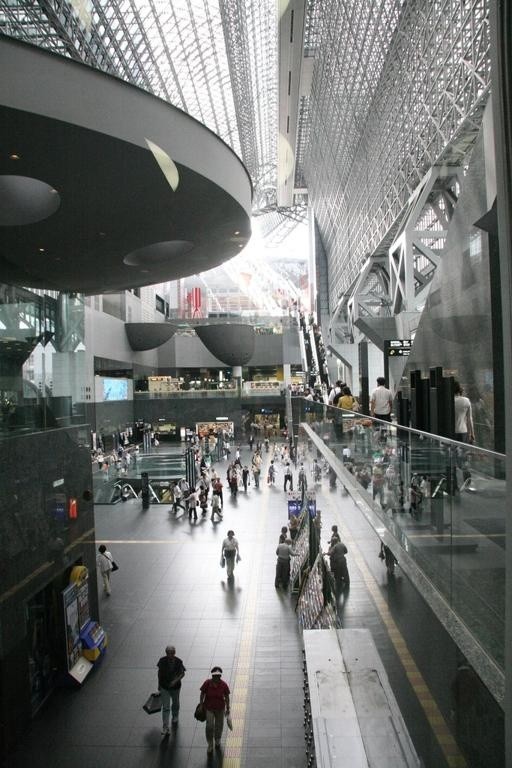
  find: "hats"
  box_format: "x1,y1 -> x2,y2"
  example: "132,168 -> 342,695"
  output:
211,669 -> 223,677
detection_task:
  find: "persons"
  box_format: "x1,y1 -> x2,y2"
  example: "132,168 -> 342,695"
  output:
156,645 -> 188,736
221,530 -> 239,580
46,532 -> 64,561
316,510 -> 351,590
275,514 -> 301,589
88,408 -> 306,522
197,666 -> 231,754
466,378 -> 496,474
135,308 -> 329,395
96,544 -> 114,596
306,377 -> 477,522
380,542 -> 398,576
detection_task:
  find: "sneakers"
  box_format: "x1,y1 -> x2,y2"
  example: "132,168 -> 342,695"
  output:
207,738 -> 221,753
161,720 -> 178,735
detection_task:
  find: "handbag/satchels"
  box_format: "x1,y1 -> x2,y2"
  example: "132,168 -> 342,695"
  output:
220,555 -> 225,568
142,690 -> 163,715
193,702 -> 207,722
111,562 -> 119,572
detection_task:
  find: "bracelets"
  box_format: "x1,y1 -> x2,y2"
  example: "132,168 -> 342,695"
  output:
226,707 -> 229,709
